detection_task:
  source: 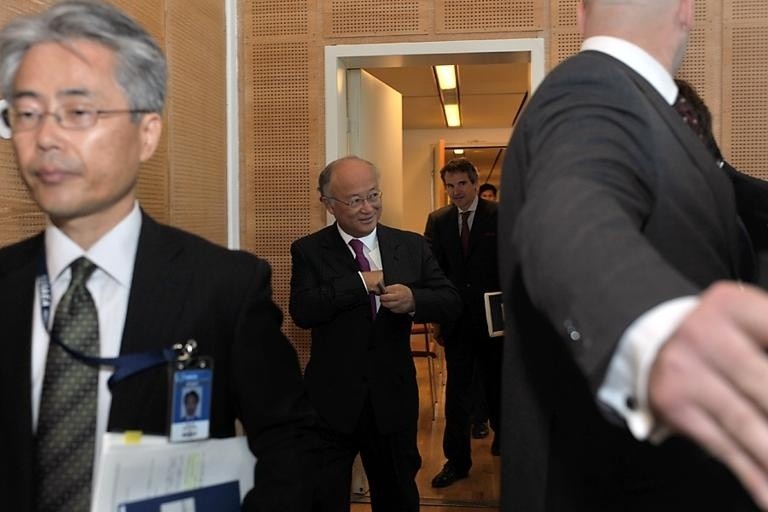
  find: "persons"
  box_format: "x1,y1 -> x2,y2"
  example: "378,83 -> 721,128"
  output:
178,387 -> 200,421
663,77 -> 766,289
0,0 -> 314,512
477,182 -> 496,206
471,411 -> 490,438
494,0 -> 768,512
424,156 -> 501,492
285,154 -> 462,512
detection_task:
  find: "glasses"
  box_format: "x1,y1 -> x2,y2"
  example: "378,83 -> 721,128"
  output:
1,96 -> 152,141
327,190 -> 384,209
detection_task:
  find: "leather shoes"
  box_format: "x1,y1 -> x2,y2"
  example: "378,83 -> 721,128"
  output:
431,458 -> 470,489
473,419 -> 502,458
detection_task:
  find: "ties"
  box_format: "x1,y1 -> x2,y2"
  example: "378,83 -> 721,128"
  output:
460,211 -> 473,260
349,240 -> 378,318
33,256 -> 103,512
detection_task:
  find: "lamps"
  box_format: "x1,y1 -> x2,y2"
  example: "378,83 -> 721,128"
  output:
431,65 -> 461,127
